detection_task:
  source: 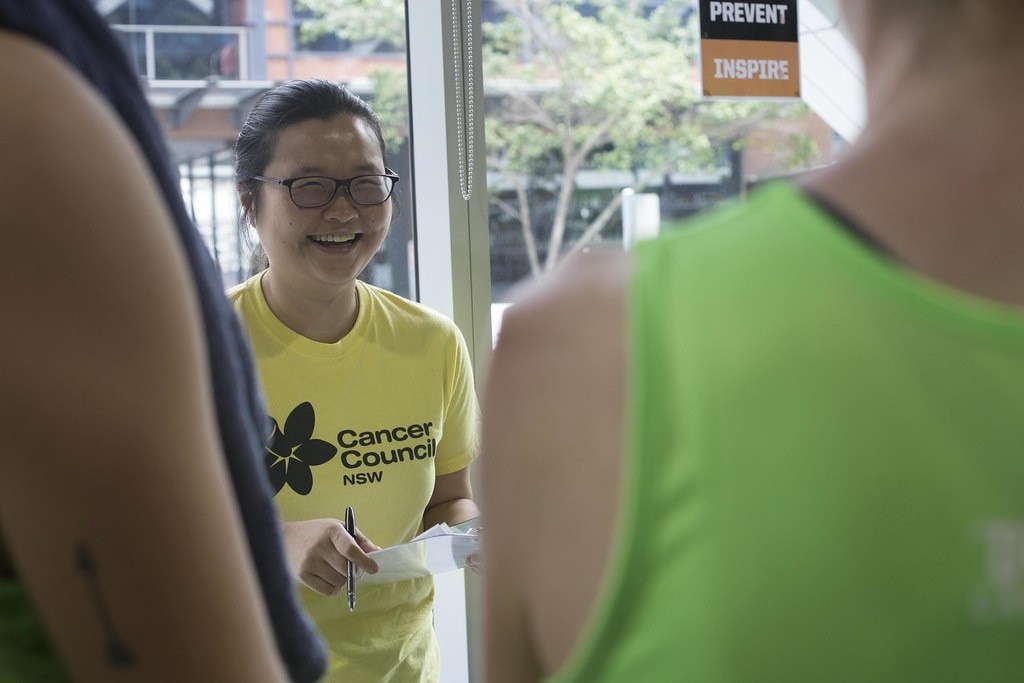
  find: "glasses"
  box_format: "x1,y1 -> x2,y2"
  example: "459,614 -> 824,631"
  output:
248,167 -> 400,209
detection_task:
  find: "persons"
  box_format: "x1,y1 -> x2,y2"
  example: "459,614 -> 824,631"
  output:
1,0 -> 332,682
477,0 -> 1024,683
221,75 -> 486,683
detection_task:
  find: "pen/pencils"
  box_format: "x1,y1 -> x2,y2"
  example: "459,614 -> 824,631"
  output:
343,507 -> 357,612
